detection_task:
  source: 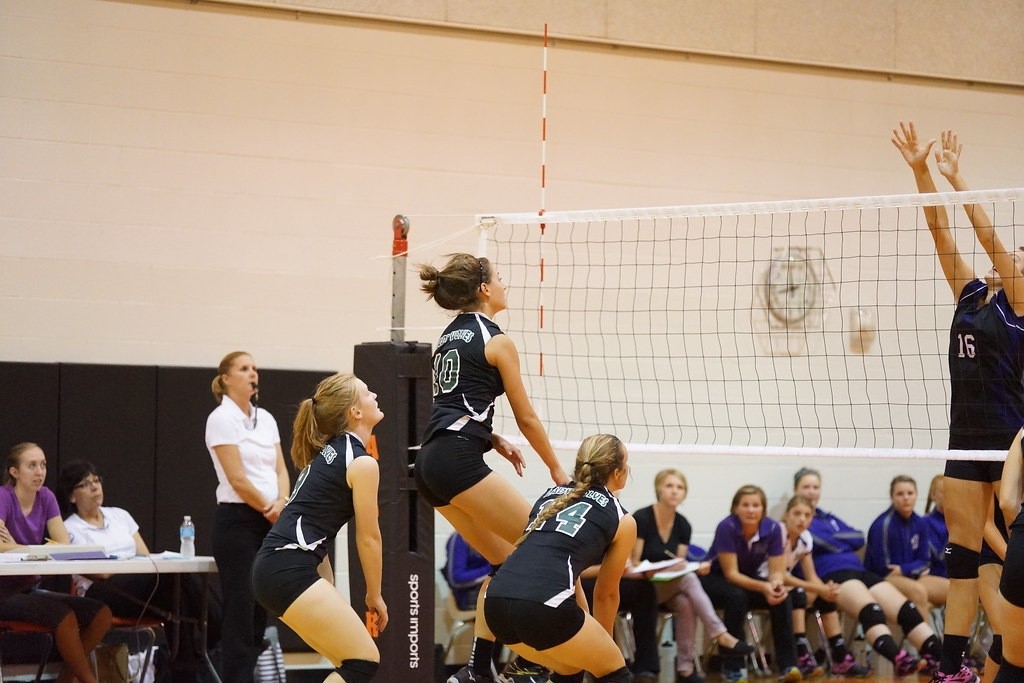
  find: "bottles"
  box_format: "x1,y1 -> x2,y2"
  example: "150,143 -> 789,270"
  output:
180,515 -> 196,557
659,640 -> 675,682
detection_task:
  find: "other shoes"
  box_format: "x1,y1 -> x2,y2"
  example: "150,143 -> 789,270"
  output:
721,662 -> 749,683
635,643 -> 660,677
718,638 -> 750,656
778,666 -> 802,683
677,674 -> 706,683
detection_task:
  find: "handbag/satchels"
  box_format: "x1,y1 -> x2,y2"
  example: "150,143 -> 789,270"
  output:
86,643 -> 129,683
256,627 -> 286,683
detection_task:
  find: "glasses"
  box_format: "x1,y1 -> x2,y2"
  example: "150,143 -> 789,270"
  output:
73,475 -> 104,488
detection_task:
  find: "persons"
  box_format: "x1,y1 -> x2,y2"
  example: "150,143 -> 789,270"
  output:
632,470 -> 755,683
697,485 -> 803,683
204,352 -> 291,683
483,433 -> 637,683
249,373 -> 388,683
865,121 -> 1024,683
413,254 -> 570,683
776,495 -> 876,679
439,529 -> 661,683
0,441 -> 113,683
47,458 -> 179,632
795,468 -> 975,679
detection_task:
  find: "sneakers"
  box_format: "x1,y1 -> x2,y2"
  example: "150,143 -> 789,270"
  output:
831,653 -> 875,678
496,658 -> 554,683
797,653 -> 824,678
894,647 -> 928,679
923,653 -> 985,683
447,667 -> 495,683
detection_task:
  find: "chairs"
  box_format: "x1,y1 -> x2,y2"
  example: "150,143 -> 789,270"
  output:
441,589 -> 990,678
0,613 -> 174,682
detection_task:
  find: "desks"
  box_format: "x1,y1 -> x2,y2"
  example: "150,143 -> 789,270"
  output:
0,553 -> 223,683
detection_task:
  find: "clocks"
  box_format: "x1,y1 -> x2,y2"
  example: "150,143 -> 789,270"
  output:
765,254 -> 817,324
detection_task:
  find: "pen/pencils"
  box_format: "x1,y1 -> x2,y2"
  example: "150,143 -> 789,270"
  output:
45,538 -> 58,544
